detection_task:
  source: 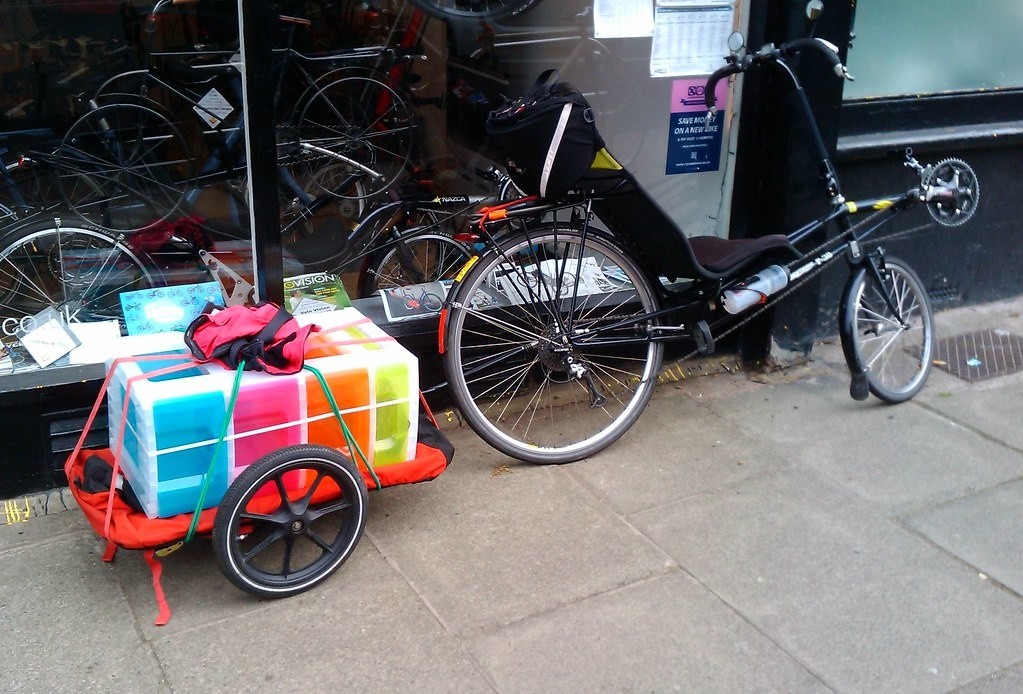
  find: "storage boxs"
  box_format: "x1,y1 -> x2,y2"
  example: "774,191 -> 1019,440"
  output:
59,237 -> 308,307
103,306 -> 420,520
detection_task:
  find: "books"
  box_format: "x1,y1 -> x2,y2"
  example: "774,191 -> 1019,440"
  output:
281,271 -> 354,318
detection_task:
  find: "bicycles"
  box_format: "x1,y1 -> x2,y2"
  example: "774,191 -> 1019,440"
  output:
436,0 -> 981,467
1,0 -> 558,349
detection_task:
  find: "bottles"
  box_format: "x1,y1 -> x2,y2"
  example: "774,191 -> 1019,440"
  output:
721,264 -> 792,314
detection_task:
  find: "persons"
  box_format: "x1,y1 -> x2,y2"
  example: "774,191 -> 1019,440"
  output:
287,289 -> 302,309
